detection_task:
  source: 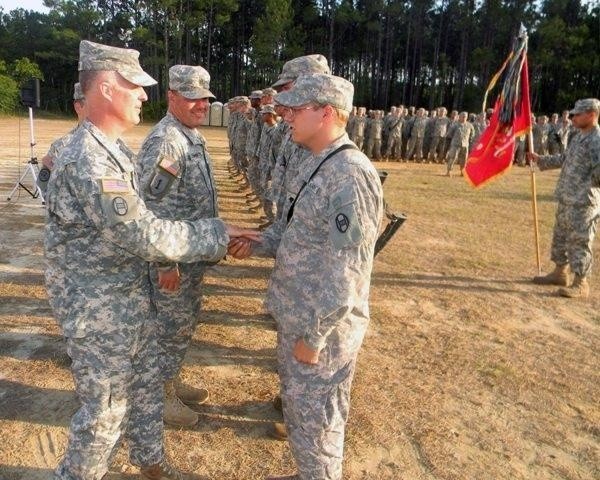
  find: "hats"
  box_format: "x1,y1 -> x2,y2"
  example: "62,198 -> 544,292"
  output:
271,54 -> 330,88
352,105 -> 494,118
568,98 -> 600,115
168,65 -> 218,100
226,88 -> 277,115
73,82 -> 85,100
273,73 -> 354,114
532,110 -> 569,121
78,40 -> 159,88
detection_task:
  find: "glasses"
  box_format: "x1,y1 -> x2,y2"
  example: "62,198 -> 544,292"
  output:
288,105 -> 324,118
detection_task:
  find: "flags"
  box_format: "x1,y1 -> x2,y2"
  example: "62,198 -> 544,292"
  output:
461,22 -> 533,188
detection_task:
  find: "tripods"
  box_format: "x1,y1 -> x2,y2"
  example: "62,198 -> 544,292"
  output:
6,107 -> 45,205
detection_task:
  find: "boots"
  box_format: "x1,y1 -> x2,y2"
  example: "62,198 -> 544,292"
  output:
162,375 -> 209,427
446,169 -> 464,177
272,392 -> 283,410
377,156 -> 445,164
227,158 -> 273,230
533,262 -> 571,286
267,418 -> 289,441
558,273 -> 589,298
142,457 -> 205,479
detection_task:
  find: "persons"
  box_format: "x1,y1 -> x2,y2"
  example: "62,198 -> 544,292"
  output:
345,105 -> 575,178
526,99 -> 600,298
226,55 -> 384,480
137,64 -> 218,428
43,39 -> 265,480
36,83 -> 136,203
